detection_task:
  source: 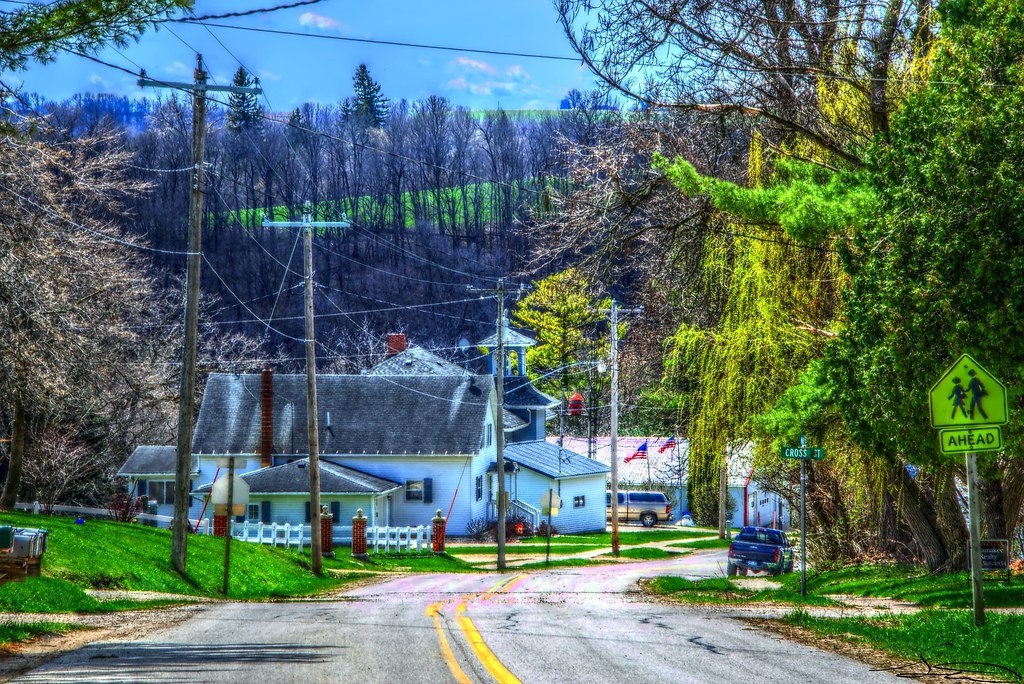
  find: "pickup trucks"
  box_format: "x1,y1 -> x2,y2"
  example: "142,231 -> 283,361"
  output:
725,526 -> 795,577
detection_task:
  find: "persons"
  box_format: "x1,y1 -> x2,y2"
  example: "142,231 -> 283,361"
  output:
32,496 -> 42,514
74,513 -> 85,525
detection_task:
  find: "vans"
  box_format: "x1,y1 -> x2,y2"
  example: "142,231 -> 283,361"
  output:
605,490 -> 675,528
